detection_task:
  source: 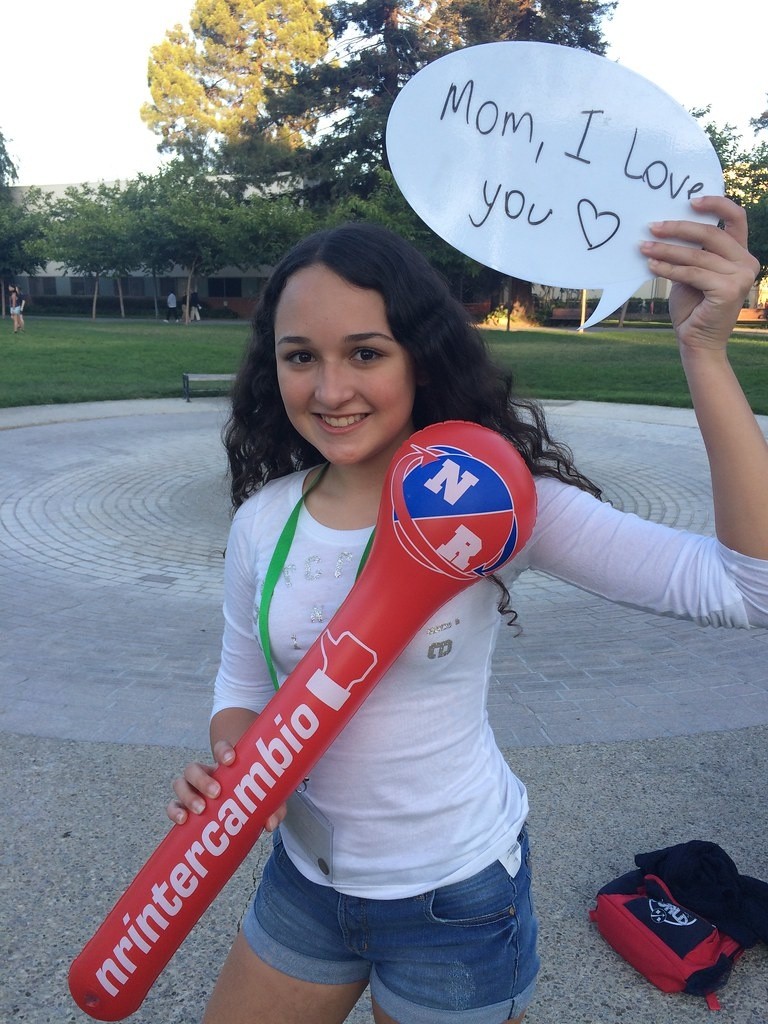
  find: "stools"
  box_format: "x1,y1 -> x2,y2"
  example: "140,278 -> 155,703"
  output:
182,372 -> 237,402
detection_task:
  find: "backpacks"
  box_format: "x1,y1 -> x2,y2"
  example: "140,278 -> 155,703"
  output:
587,840 -> 768,1011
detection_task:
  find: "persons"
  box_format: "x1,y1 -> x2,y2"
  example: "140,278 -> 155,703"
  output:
182,290 -> 202,322
165,195 -> 767,1024
8,284 -> 26,333
162,290 -> 179,324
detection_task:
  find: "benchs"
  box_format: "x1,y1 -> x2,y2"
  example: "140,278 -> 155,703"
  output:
463,303 -> 490,319
548,308 -> 593,326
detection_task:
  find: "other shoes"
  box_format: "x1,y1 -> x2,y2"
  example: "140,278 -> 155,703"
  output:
14,330 -> 17,334
163,320 -> 169,323
19,328 -> 25,334
175,319 -> 179,323
